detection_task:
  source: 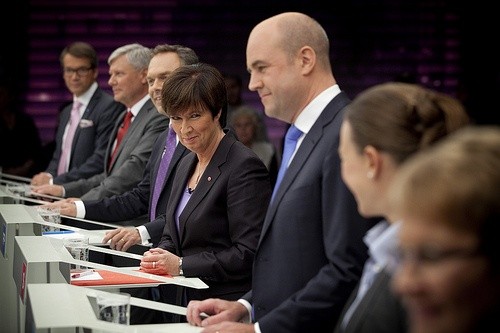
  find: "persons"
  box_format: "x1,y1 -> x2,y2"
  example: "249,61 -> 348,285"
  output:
185,11 -> 384,333
20,41 -> 171,223
37,40 -> 240,256
332,78 -> 500,333
225,74 -> 279,191
139,62 -> 270,311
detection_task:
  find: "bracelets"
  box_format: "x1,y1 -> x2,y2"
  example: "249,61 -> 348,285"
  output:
179,257 -> 183,276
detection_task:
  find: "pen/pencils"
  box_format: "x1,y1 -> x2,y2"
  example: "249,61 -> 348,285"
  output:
43,231 -> 75,234
71,270 -> 95,278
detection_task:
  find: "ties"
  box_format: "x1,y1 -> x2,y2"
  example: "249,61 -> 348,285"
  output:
150,125 -> 176,223
270,124 -> 303,206
57,99 -> 82,176
110,110 -> 135,167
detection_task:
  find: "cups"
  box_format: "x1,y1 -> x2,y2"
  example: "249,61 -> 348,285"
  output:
7,182 -> 25,204
37,204 -> 60,232
62,235 -> 89,273
96,292 -> 130,327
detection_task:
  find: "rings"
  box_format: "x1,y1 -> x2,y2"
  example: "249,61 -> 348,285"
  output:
121,238 -> 127,243
153,261 -> 156,269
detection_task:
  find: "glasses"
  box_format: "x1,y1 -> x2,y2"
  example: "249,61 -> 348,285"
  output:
388,245 -> 478,262
64,66 -> 94,77
